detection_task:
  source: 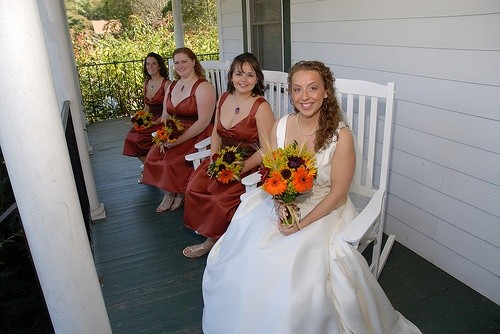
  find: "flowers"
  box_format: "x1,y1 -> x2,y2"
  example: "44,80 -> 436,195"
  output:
206,145 -> 245,184
152,116 -> 186,144
255,139 -> 318,226
130,111 -> 153,131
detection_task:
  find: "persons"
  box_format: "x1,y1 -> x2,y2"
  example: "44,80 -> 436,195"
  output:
182,52 -> 276,259
201,60 -> 361,334
142,47 -> 217,213
122,52 -> 173,184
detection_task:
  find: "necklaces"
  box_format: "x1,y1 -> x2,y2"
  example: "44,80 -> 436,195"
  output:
296,114 -> 318,136
234,92 -> 250,114
151,86 -> 153,90
180,85 -> 184,92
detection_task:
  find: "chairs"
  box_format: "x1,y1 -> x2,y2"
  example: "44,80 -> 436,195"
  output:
148,59 -> 396,281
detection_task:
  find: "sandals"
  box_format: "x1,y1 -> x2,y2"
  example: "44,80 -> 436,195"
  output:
171,195 -> 186,211
156,196 -> 175,212
183,243 -> 208,258
